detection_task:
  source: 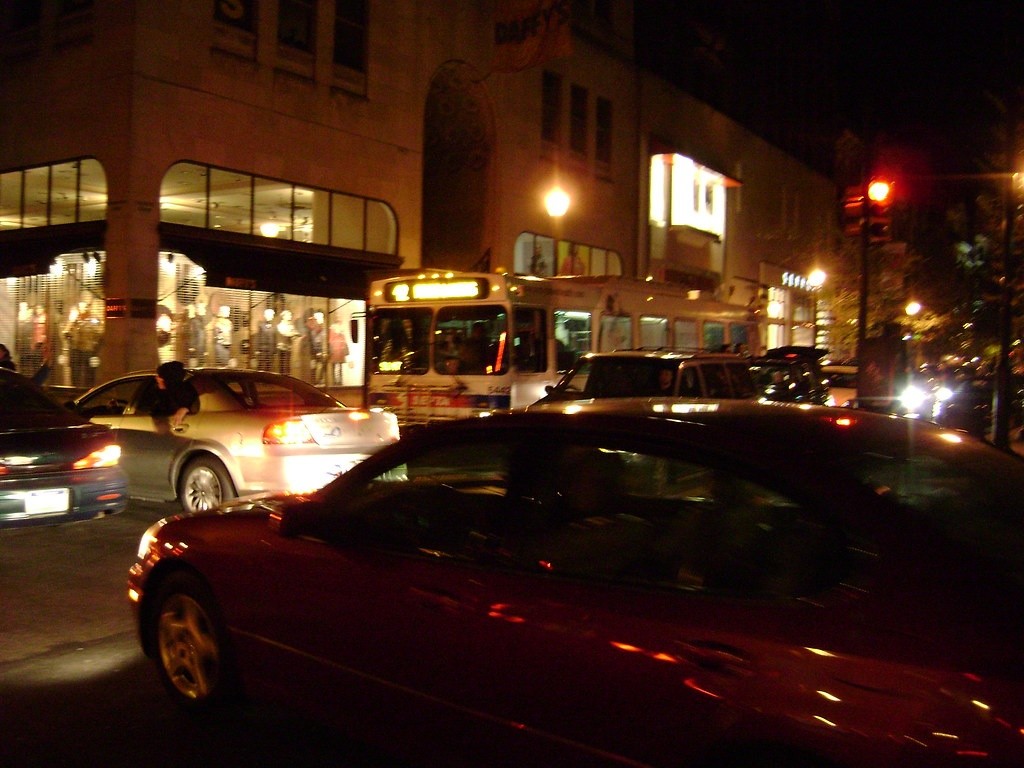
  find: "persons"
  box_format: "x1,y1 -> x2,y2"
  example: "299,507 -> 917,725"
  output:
561,244 -> 586,277
15,289 -> 349,390
146,361 -> 198,427
0,344 -> 15,371
646,323 -> 1024,458
530,245 -> 547,278
107,398 -> 119,415
376,318 -> 570,376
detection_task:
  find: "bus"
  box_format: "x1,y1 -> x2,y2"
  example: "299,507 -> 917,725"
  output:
363,266 -> 762,420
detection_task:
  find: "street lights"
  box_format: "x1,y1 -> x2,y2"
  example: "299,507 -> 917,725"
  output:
856,166 -> 896,350
990,165 -> 1024,449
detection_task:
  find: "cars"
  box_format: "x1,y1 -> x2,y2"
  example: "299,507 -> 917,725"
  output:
0,369 -> 126,526
535,349 -> 758,400
747,344 -> 864,406
67,366 -> 413,516
126,397 -> 1024,768
